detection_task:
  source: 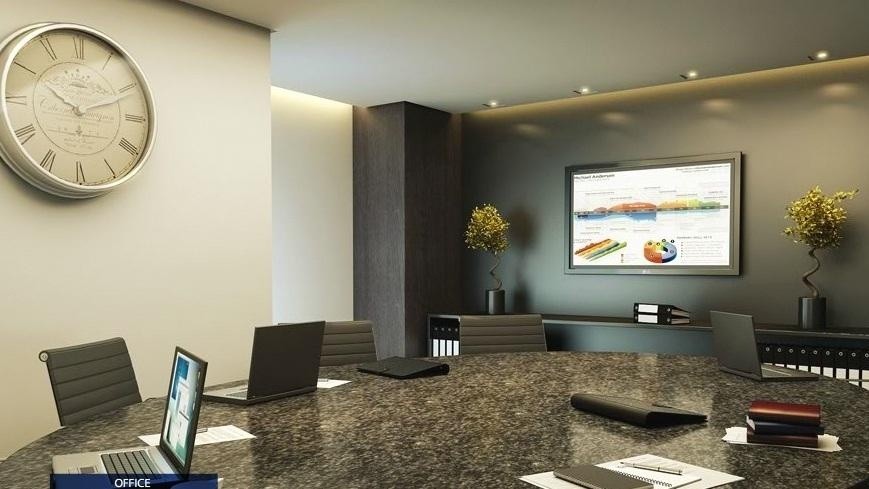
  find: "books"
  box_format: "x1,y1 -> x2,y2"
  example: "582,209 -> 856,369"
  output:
746,399 -> 825,449
633,302 -> 690,318
633,313 -> 691,324
552,454 -> 701,488
569,387 -> 710,430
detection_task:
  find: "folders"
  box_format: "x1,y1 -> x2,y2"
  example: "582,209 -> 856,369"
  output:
432,318 -> 459,356
572,392 -> 709,428
634,303 -> 690,324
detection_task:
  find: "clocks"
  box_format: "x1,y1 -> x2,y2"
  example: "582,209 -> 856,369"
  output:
0,19 -> 159,199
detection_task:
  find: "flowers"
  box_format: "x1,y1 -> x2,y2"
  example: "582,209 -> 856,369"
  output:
461,203 -> 511,290
781,184 -> 862,298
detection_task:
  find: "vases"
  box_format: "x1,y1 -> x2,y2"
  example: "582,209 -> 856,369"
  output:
484,289 -> 507,316
797,295 -> 828,330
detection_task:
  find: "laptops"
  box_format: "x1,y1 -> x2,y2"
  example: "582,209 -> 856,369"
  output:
709,309 -> 819,382
51,345 -> 209,489
202,320 -> 326,406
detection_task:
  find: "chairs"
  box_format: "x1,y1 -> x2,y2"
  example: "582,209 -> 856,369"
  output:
278,317 -> 381,371
35,332 -> 143,430
456,315 -> 549,355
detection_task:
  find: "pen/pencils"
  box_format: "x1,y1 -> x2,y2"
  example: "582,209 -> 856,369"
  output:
620,461 -> 683,475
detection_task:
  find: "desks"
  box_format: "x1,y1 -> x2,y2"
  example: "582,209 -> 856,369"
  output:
455,311 -> 868,349
0,347 -> 868,489
